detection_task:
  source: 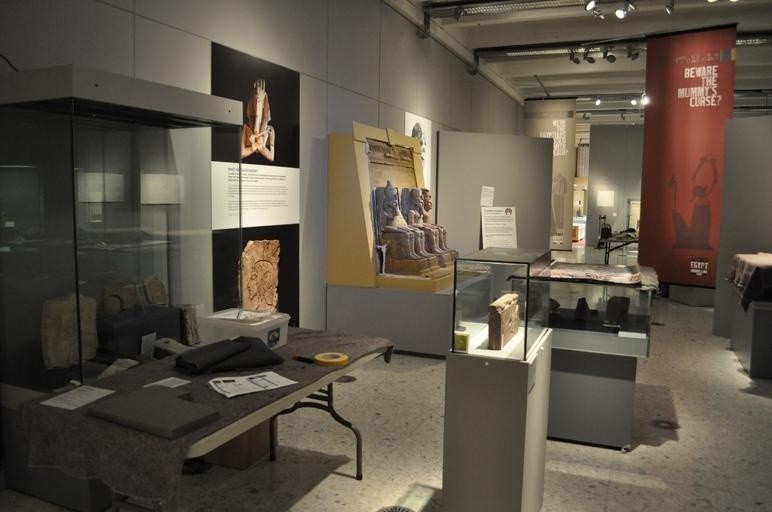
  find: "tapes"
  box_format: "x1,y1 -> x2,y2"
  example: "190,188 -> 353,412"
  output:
315,352 -> 350,366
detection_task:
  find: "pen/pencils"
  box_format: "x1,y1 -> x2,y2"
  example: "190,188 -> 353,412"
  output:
292,356 -> 313,363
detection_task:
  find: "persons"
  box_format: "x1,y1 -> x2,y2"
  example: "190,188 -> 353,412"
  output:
406,189 -> 445,254
248,260 -> 276,311
379,180 -> 423,261
421,188 -> 452,252
240,78 -> 275,161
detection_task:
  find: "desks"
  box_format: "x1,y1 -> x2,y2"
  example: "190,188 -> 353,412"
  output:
22,323 -> 392,512
